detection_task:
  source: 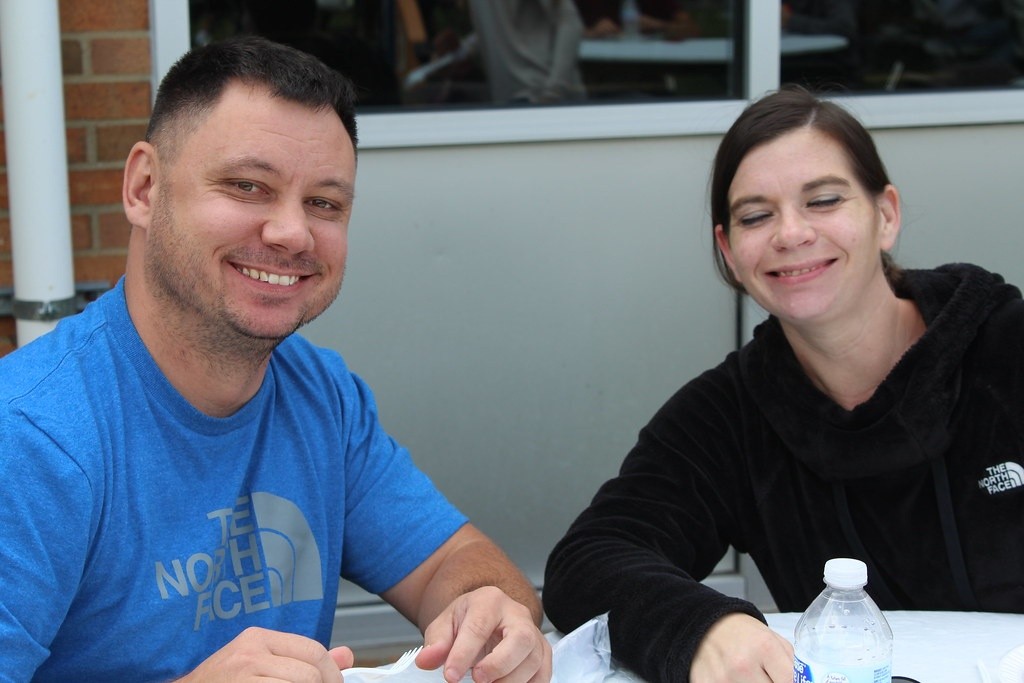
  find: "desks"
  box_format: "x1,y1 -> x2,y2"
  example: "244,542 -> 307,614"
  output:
590,35 -> 849,109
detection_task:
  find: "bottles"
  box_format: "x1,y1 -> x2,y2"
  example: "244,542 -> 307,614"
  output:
621,0 -> 639,37
792,557 -> 895,683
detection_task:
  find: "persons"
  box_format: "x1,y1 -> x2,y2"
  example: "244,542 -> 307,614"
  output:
435,0 -> 856,110
0,34 -> 553,683
541,83 -> 1024,683
